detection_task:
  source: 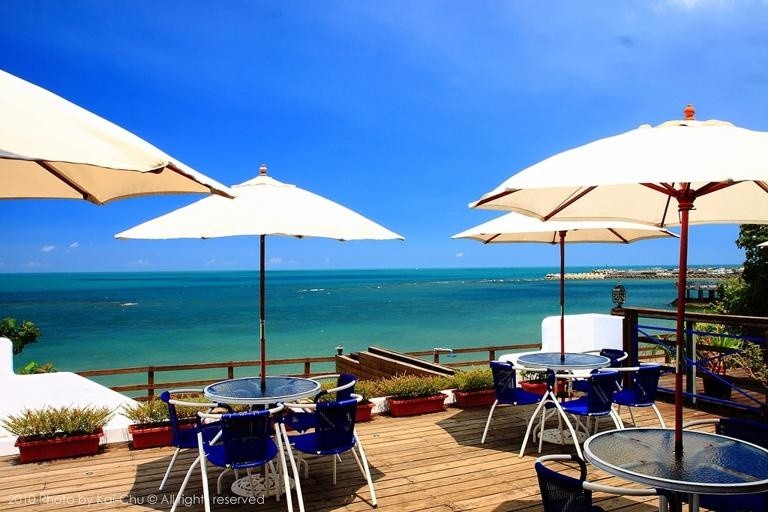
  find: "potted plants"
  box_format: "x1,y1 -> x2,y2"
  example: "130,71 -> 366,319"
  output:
637,332 -> 684,363
118,393 -> 248,451
453,365 -> 497,410
0,401 -> 122,464
374,369 -> 454,418
701,334 -> 742,401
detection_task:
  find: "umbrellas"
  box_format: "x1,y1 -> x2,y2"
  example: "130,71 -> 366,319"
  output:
470,104 -> 768,512
0,70 -> 238,204
450,209 -> 680,444
114,164 -> 404,500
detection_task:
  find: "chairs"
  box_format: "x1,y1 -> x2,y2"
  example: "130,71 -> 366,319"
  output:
676,418 -> 768,511
481,348 -> 665,458
157,373 -> 379,511
534,454 -> 681,512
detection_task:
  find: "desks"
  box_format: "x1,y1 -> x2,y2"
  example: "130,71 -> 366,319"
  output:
581,428 -> 768,512
517,353 -> 611,445
